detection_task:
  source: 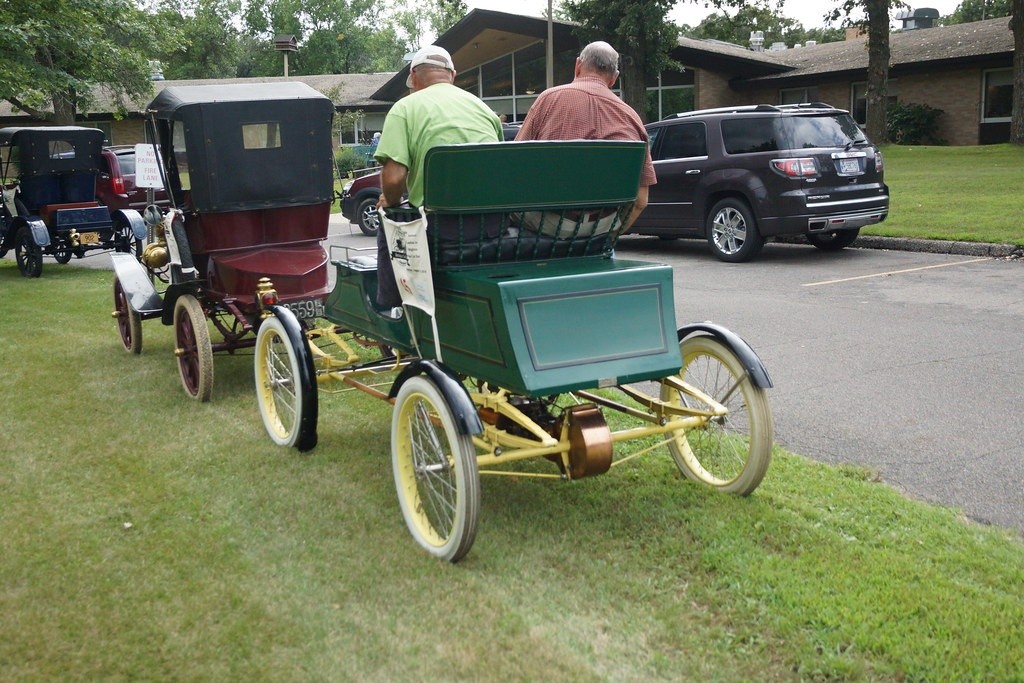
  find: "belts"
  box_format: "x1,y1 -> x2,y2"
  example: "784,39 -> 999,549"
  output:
553,207 -> 616,224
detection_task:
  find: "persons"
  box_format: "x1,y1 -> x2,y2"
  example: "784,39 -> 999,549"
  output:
373,40 -> 507,311
508,42 -> 657,235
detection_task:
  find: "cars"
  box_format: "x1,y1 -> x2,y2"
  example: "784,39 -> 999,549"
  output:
95,143 -> 174,214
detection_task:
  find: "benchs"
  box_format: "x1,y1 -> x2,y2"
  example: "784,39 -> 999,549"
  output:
423,140 -> 648,269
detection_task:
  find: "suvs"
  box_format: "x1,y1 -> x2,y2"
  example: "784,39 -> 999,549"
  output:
618,101 -> 890,264
339,121 -> 526,237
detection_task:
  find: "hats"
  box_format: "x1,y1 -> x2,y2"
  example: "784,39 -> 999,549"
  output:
406,45 -> 457,89
